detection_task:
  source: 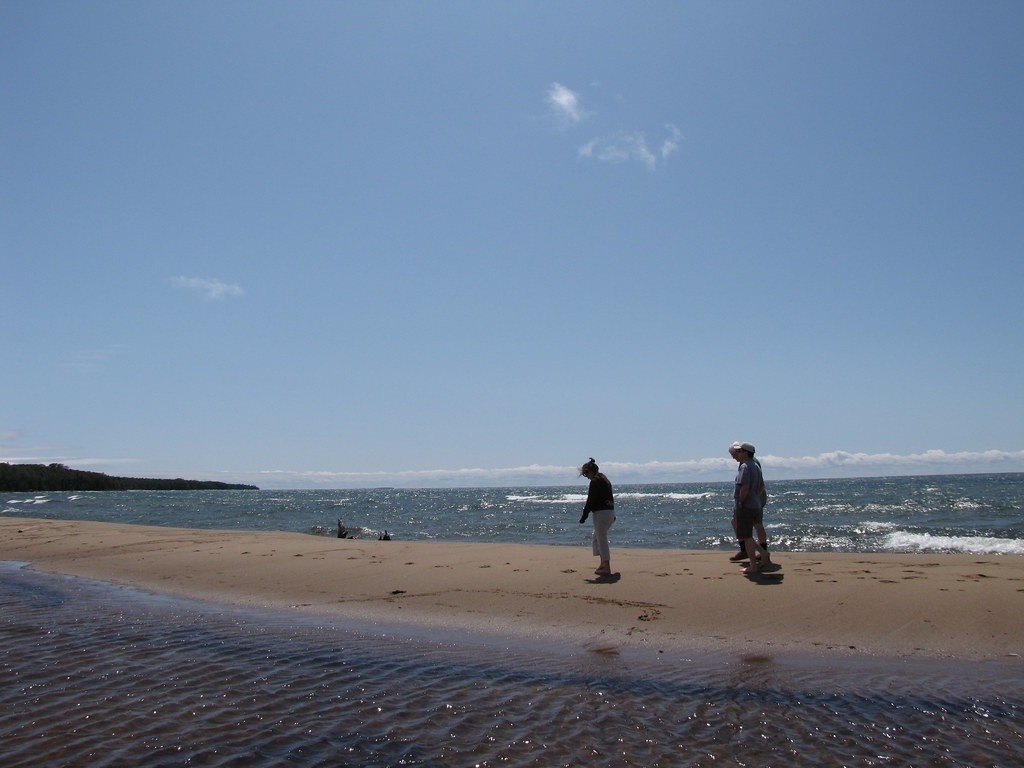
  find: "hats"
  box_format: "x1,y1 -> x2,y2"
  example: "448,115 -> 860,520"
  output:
734,442 -> 755,453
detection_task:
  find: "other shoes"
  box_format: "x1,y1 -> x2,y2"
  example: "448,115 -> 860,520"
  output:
758,551 -> 770,568
729,552 -> 748,561
756,551 -> 771,560
740,566 -> 756,573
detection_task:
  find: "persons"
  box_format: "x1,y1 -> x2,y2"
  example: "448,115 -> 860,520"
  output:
578,457 -> 616,582
728,441 -> 771,573
378,529 -> 391,541
